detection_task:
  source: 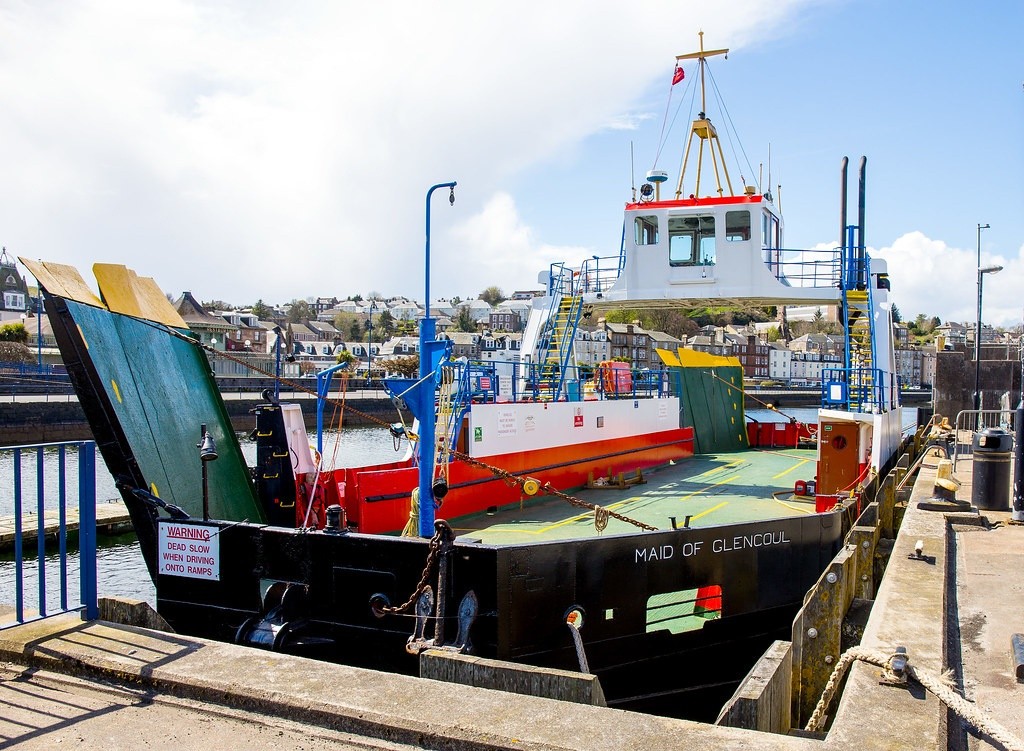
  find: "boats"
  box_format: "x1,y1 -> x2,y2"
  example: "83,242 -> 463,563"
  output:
18,31 -> 910,728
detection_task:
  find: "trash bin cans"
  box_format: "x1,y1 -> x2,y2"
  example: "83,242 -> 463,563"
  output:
971,429 -> 1013,511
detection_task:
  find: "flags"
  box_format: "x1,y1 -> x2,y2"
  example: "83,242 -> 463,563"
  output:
672,67 -> 684,85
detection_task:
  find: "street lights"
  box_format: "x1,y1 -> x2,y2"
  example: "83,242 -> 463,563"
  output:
974,265 -> 1003,430
368,302 -> 380,387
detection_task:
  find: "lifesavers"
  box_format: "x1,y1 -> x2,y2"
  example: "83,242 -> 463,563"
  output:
573,271 -> 590,292
